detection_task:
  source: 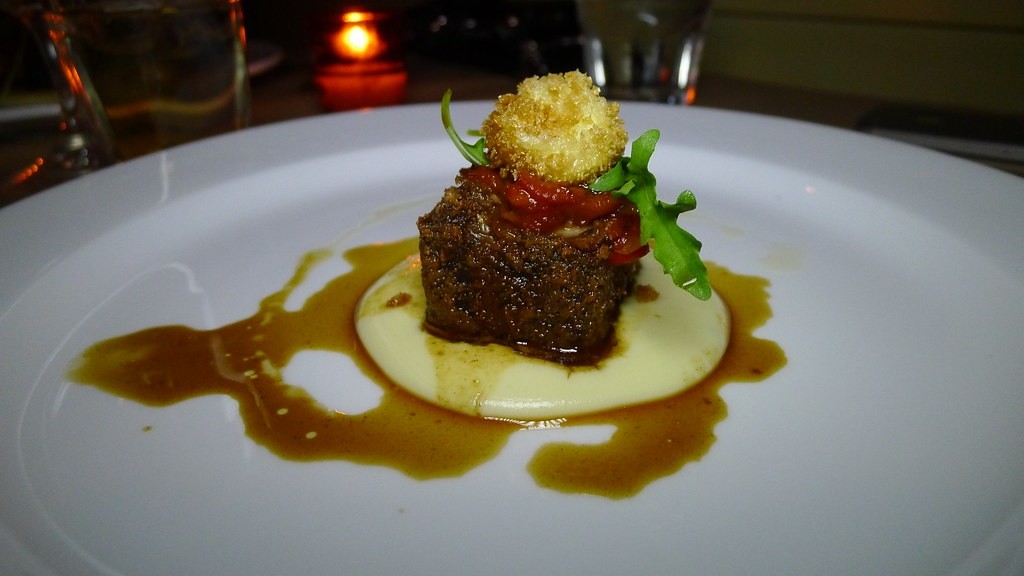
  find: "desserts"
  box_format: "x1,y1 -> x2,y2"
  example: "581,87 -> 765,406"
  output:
417,67 -> 713,368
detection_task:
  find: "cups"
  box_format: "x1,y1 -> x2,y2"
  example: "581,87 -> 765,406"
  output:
577,0 -> 714,108
1,1 -> 117,209
70,0 -> 252,162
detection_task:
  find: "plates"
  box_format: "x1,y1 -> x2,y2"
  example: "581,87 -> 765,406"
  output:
0,98 -> 1024,576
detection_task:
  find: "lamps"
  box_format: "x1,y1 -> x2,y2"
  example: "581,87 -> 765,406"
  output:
306,5 -> 414,109
